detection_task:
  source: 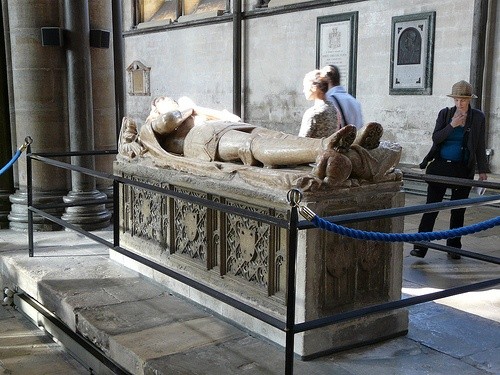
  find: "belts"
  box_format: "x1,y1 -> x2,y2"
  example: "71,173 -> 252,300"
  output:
446,159 -> 452,163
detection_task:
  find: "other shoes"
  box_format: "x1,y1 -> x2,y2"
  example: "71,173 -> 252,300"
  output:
447,253 -> 461,259
410,249 -> 427,258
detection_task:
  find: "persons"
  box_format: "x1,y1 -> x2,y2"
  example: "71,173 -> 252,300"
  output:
298,70 -> 339,138
138,97 -> 384,166
410,80 -> 491,259
321,65 -> 364,132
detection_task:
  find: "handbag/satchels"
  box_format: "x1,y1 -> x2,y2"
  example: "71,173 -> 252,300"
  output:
425,158 -> 462,188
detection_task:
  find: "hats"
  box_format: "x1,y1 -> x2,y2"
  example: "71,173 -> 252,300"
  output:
446,80 -> 479,98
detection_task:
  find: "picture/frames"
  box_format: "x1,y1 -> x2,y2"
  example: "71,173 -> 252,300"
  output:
315,10 -> 359,99
390,10 -> 436,97
